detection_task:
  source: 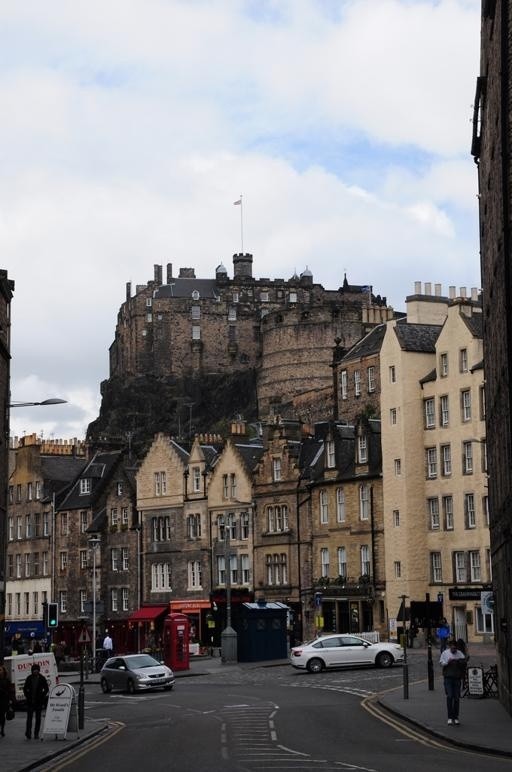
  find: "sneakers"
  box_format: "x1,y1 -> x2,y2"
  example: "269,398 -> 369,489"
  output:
447,718 -> 460,726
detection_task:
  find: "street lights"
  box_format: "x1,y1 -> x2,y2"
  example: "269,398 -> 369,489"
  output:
88,539 -> 103,672
0,398 -> 69,658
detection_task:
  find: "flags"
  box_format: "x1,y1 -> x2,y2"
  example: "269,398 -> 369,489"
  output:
233,200 -> 242,205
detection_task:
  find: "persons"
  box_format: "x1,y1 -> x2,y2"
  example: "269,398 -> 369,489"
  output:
95,634 -> 105,649
1,665 -> 15,736
438,640 -> 467,727
103,634 -> 113,659
436,617 -> 451,653
455,638 -> 467,656
4,635 -> 65,666
23,663 -> 50,740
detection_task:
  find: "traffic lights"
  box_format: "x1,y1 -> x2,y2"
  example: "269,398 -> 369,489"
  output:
47,603 -> 59,628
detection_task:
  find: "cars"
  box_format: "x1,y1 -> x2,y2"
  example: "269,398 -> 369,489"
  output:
100,654 -> 175,694
287,633 -> 405,674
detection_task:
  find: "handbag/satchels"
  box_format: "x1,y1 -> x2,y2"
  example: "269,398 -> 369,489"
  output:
6,709 -> 15,721
